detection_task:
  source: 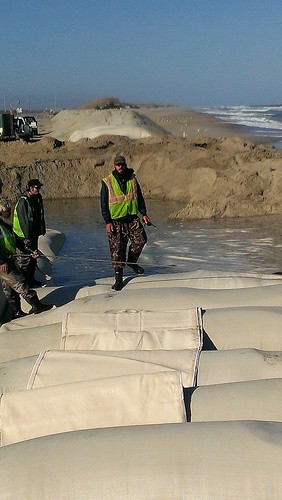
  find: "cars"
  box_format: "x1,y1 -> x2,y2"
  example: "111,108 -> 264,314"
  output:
0,114 -> 38,140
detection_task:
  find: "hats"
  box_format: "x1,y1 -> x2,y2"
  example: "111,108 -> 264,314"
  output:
114,156 -> 126,164
28,179 -> 43,187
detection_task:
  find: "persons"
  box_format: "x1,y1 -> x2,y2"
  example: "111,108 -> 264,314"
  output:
12,179 -> 46,286
100,156 -> 152,291
0,203 -> 53,318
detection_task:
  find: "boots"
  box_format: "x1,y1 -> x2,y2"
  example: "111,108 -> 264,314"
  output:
13,308 -> 27,319
111,275 -> 123,291
26,273 -> 46,288
127,256 -> 145,274
24,290 -> 53,314
